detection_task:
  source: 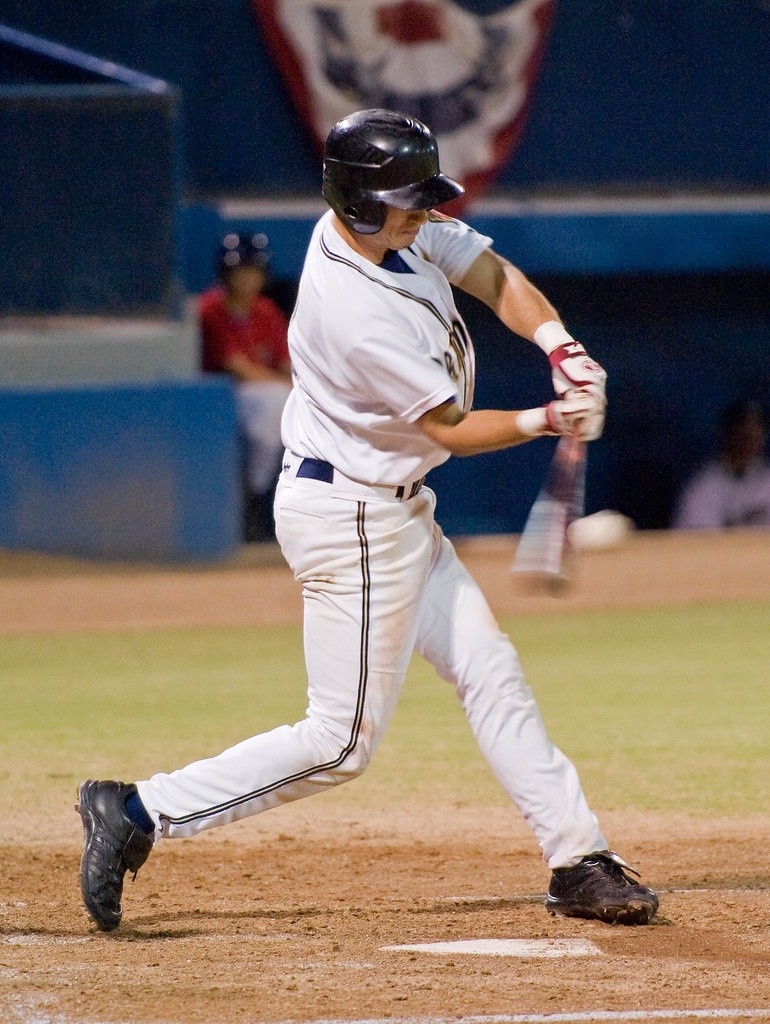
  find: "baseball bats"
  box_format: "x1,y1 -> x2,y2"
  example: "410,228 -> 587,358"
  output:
510,417 -> 588,599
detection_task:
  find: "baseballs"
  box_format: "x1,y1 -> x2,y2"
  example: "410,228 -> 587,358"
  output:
566,509 -> 634,555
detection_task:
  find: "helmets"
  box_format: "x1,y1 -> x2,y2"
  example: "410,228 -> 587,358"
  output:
217,233 -> 270,292
321,108 -> 466,234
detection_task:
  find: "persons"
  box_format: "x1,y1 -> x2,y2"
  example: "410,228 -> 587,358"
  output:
674,400 -> 770,529
194,230 -> 292,524
75,108 -> 659,930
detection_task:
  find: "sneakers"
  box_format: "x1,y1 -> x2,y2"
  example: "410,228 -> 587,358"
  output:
544,850 -> 659,927
73,777 -> 155,931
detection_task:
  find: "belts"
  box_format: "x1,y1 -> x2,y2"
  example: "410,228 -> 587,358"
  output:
296,458 -> 427,499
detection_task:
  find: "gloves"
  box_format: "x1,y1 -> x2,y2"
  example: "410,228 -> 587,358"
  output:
547,340 -> 608,407
538,397 -> 605,442
